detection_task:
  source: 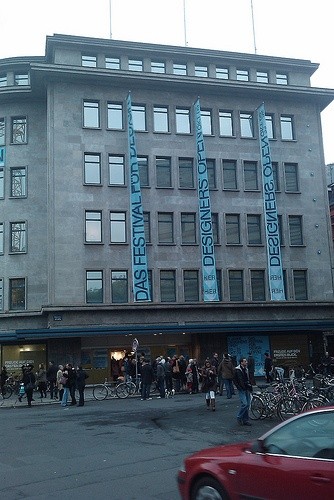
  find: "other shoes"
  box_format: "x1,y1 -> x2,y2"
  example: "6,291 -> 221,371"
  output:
237,418 -> 244,426
244,421 -> 252,426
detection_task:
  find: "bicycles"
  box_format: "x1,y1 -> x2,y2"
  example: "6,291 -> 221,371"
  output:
247,358 -> 334,423
93,376 -> 131,401
0,372 -> 55,405
115,374 -> 167,395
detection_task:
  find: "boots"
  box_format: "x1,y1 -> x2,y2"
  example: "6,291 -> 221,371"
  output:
211,399 -> 216,410
206,399 -> 211,410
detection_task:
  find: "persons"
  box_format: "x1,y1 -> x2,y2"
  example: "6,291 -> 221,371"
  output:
200,356 -> 217,412
246,352 -> 256,385
37,363 -> 48,398
1,365 -> 8,395
47,360 -> 60,399
233,357 -> 254,427
264,353 -> 273,382
57,365 -> 65,400
61,365 -> 75,406
19,364 -> 36,402
62,363 -> 78,406
22,368 -> 36,407
112,351 -> 237,400
75,365 -> 90,407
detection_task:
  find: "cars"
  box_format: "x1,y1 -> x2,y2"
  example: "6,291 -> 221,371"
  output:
177,406 -> 334,500
